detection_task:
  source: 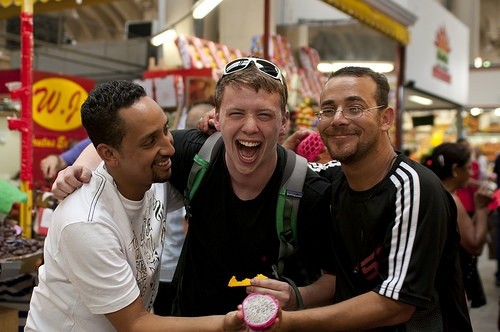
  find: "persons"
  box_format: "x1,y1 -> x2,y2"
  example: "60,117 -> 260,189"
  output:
238,67 -> 473,332
24,80 -> 282,332
40,55 -> 500,332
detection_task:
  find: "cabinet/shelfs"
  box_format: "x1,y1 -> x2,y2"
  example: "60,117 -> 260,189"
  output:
388,107 -> 499,166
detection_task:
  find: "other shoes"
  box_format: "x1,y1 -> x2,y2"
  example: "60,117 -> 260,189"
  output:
470,298 -> 485,307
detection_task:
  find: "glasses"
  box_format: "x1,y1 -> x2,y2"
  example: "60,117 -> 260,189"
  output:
221,58 -> 283,85
316,105 -> 388,118
